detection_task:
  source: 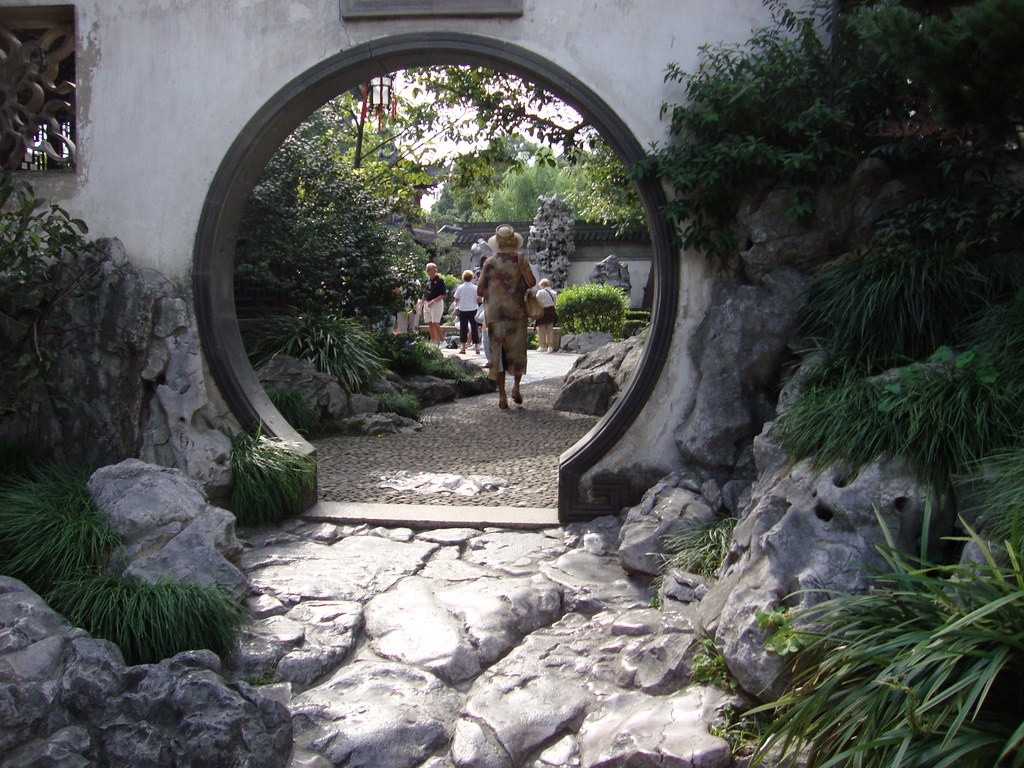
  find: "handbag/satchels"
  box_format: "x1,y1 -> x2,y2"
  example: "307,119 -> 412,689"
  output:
474,304 -> 485,323
517,252 -> 545,319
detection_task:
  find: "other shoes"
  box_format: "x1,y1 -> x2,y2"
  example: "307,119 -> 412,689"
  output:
547,347 -> 553,354
498,400 -> 509,412
511,389 -> 522,404
536,346 -> 544,352
459,350 -> 466,354
481,362 -> 490,368
475,349 -> 480,354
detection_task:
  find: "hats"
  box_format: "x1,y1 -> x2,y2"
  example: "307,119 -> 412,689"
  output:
488,224 -> 524,252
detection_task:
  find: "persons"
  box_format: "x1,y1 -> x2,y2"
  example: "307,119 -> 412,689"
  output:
454,271 -> 480,354
374,263 -> 447,349
474,271 -> 491,368
536,279 -> 555,353
477,224 -> 536,407
